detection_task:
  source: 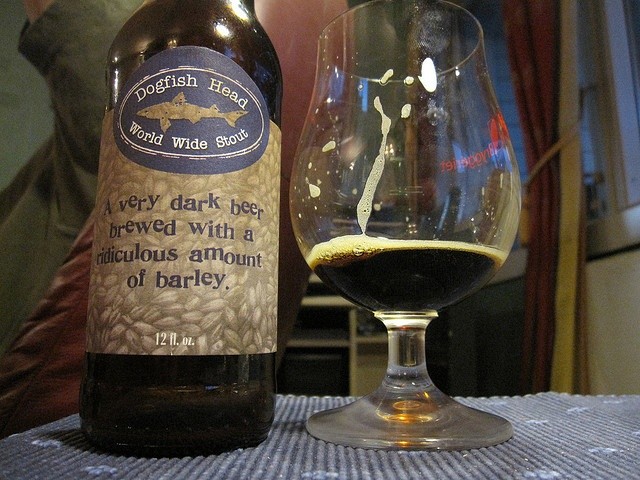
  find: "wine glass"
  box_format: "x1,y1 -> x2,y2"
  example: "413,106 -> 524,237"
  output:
289,0 -> 522,452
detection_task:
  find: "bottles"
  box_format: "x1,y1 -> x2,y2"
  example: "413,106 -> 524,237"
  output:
79,1 -> 282,457
